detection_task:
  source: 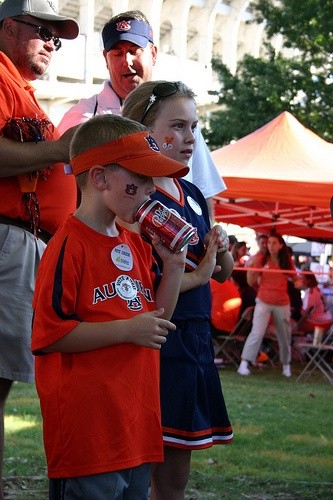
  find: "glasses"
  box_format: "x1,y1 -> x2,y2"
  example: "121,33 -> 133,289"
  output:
12,18 -> 61,51
140,81 -> 187,124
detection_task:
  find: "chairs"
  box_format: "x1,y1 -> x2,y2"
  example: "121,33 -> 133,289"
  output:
295,326 -> 333,385
264,305 -> 315,369
213,307 -> 254,370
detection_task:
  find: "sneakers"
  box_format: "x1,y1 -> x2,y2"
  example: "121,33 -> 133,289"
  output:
283,364 -> 291,377
237,360 -> 250,376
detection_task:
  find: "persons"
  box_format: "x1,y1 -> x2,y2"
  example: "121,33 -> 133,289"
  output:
122,80 -> 235,500
56,10 -> 228,222
0,0 -> 79,500
209,276 -> 242,369
228,234 -> 333,368
238,233 -> 302,377
31,113 -> 190,500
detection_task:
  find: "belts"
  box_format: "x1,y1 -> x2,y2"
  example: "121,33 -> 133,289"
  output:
0,214 -> 54,245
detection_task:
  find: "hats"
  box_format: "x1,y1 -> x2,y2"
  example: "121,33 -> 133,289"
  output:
0,0 -> 79,39
64,131 -> 190,178
102,18 -> 154,53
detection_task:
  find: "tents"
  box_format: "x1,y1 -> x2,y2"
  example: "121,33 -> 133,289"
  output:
210,111 -> 333,238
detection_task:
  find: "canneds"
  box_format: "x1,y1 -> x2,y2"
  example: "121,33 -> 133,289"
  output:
134,199 -> 195,253
